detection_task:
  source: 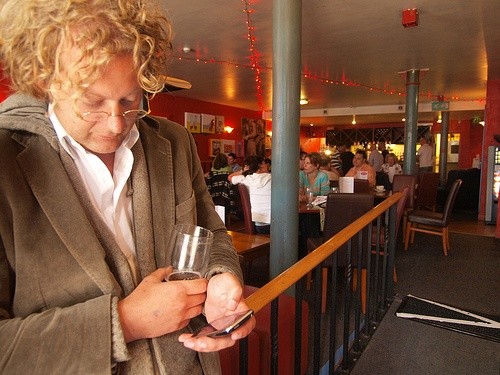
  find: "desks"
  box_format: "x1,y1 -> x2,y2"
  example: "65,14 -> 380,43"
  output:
227,230 -> 270,255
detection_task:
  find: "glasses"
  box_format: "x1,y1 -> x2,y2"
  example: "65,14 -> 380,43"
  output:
60,89 -> 151,122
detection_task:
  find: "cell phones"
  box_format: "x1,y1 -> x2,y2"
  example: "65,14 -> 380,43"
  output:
192,310 -> 254,339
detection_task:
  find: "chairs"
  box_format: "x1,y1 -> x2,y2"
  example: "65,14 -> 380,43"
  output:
235,172 -> 462,316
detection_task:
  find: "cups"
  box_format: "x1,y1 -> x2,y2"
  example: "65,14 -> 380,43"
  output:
376,186 -> 384,192
304,186 -> 313,208
163,222 -> 214,282
331,187 -> 339,193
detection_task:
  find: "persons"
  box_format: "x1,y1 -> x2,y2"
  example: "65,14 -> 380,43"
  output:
228,153 -> 240,172
418,137 -> 433,172
369,144 -> 384,182
331,144 -> 344,176
319,156 -> 341,180
341,142 -> 355,174
382,153 -> 403,183
0,0 -> 257,375
228,156 -> 271,234
212,153 -> 234,175
299,152 -> 331,201
300,151 -> 308,169
345,150 -> 377,188
